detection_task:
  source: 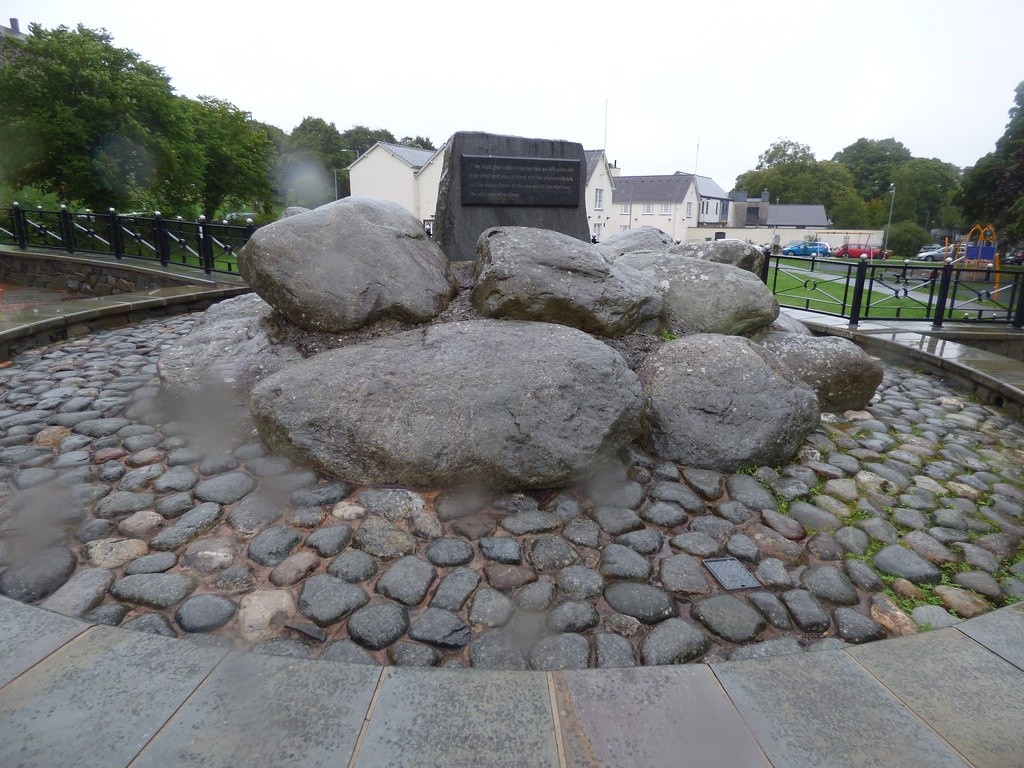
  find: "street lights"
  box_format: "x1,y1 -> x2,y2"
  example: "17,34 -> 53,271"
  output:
339,149 -> 359,159
883,182 -> 896,259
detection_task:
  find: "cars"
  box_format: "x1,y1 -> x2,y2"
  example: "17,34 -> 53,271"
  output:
917,246 -> 966,261
1005,247 -> 1024,264
922,246 -> 941,253
950,241 -> 974,248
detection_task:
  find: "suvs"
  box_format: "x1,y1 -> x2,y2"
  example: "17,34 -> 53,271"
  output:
783,241 -> 831,258
836,244 -> 889,259
